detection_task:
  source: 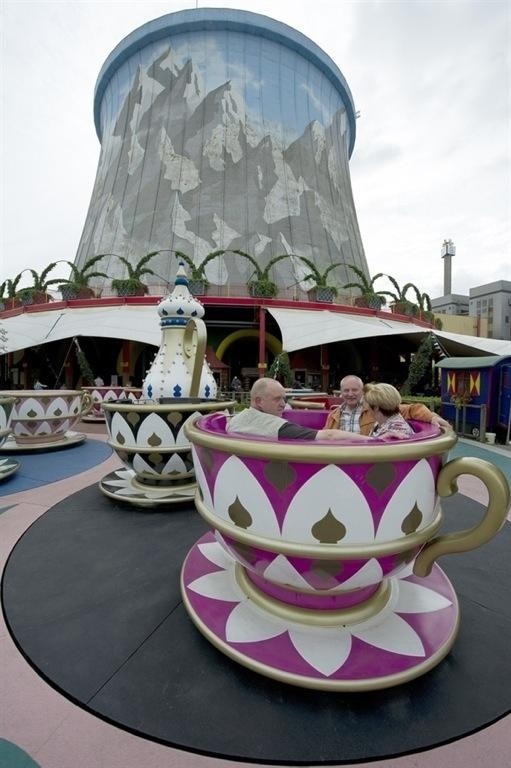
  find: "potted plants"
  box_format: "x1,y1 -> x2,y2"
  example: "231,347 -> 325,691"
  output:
1,247 -> 296,315
300,250 -> 444,329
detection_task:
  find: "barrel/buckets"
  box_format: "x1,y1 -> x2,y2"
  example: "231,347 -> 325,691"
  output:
486,433 -> 496,444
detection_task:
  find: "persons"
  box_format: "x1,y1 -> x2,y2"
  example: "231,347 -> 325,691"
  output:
225,378 -> 410,441
34,376 -> 48,390
363,383 -> 415,439
321,375 -> 453,436
231,376 -> 241,389
